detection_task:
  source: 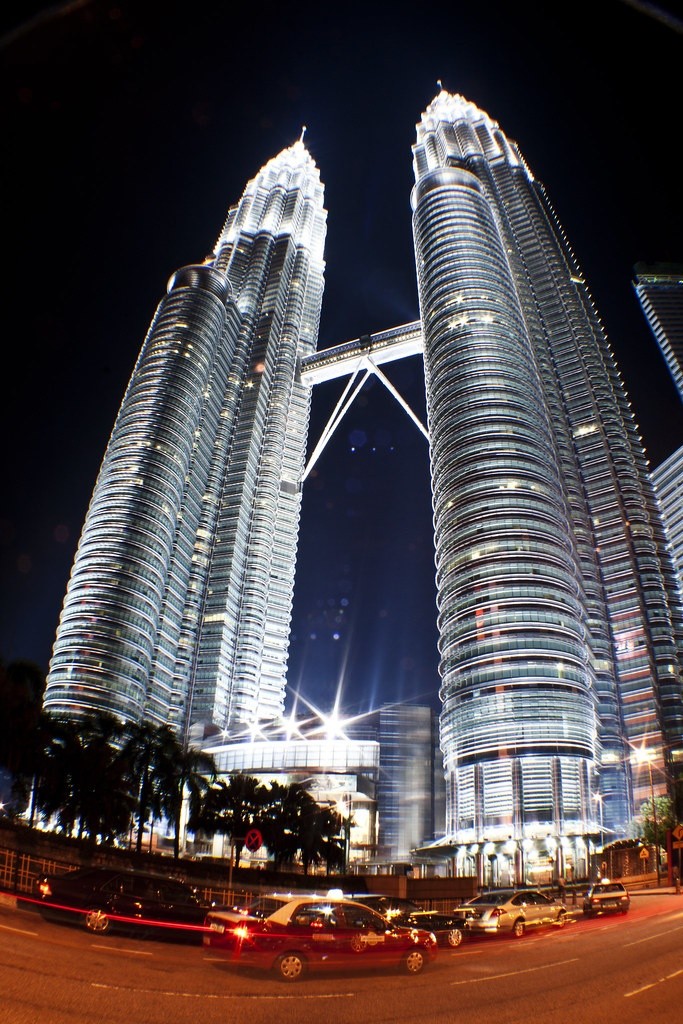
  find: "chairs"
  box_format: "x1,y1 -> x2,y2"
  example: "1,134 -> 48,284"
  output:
354,920 -> 363,927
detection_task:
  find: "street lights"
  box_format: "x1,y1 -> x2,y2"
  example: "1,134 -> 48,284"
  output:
593,795 -> 604,862
635,751 -> 662,886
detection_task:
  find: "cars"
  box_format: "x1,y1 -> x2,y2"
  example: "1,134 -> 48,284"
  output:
582,880 -> 630,914
310,893 -> 472,949
33,867 -> 235,945
202,889 -> 440,980
455,890 -> 570,939
